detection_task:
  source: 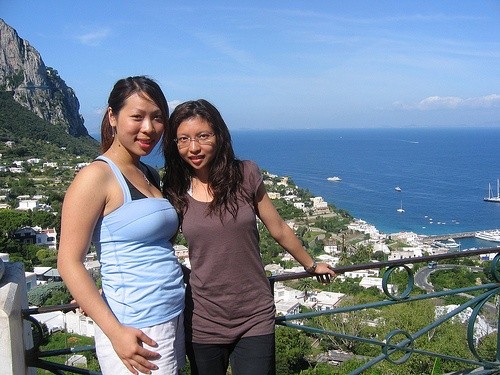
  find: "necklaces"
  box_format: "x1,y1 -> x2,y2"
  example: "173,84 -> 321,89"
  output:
112,149 -> 150,184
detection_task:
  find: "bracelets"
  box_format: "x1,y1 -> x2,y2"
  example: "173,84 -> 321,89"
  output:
306,263 -> 317,273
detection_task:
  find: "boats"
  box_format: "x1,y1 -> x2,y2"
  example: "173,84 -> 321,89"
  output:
397,209 -> 406,213
474,229 -> 500,242
328,176 -> 343,182
483,179 -> 500,202
395,187 -> 402,191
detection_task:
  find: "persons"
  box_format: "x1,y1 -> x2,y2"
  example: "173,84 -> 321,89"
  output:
57,76 -> 187,375
159,99 -> 338,375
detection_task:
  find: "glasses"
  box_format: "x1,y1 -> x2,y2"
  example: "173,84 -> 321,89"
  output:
175,131 -> 216,146
